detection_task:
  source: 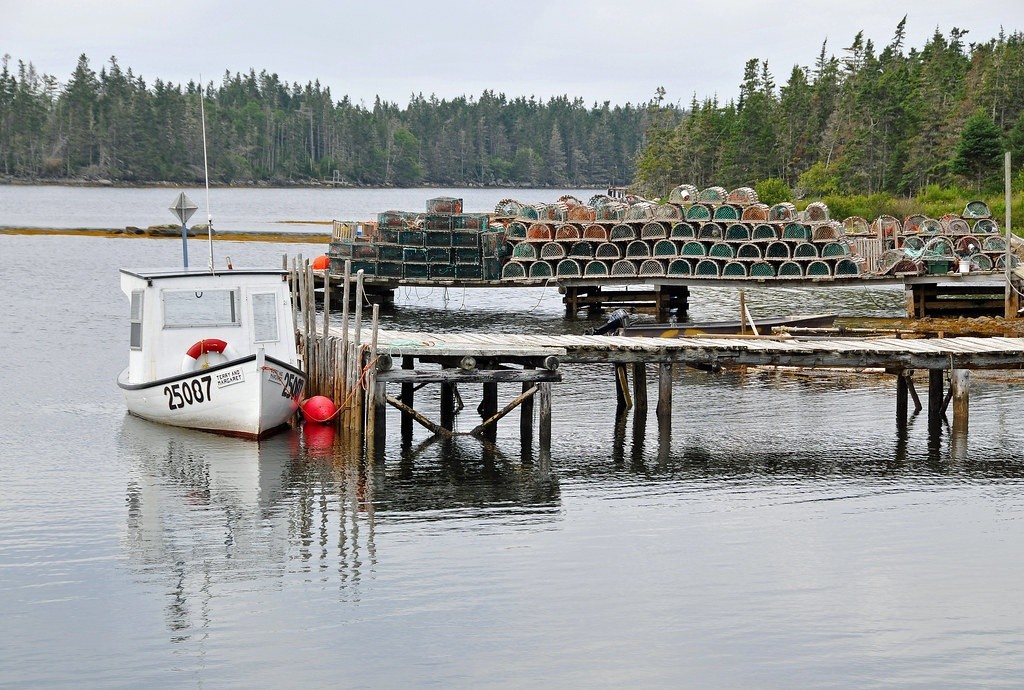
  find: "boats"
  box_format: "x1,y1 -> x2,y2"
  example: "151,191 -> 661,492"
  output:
115,71 -> 308,441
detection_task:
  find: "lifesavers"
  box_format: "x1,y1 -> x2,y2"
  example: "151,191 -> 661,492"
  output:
181,338 -> 240,376
